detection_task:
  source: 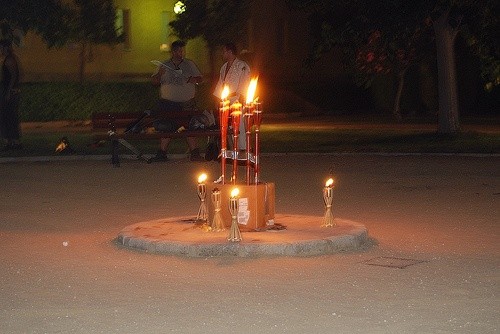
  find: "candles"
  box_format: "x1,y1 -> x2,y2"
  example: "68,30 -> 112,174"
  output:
323,177 -> 335,226
194,173 -> 243,243
219,75 -> 264,185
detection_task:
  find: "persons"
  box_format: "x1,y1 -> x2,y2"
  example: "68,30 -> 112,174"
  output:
213,43 -> 253,168
0,39 -> 23,150
151,41 -> 204,125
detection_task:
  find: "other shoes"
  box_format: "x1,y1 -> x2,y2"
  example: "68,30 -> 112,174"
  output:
147,150 -> 168,162
189,148 -> 203,160
